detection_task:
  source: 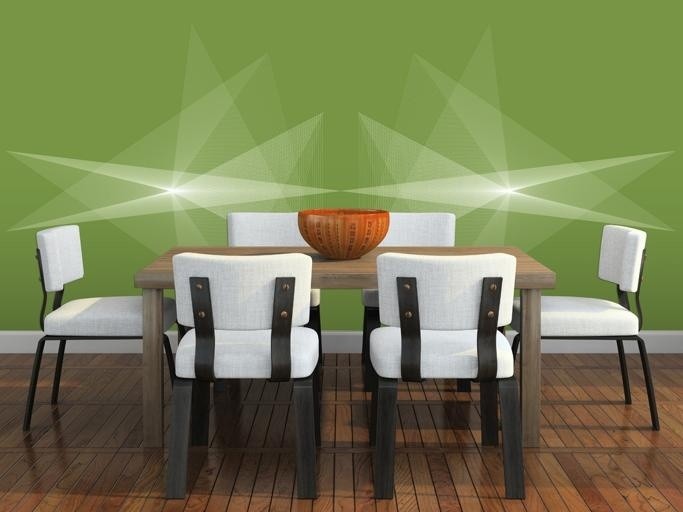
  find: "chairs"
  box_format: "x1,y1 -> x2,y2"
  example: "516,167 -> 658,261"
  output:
224,213 -> 323,399
19,222 -> 178,435
359,211 -> 457,394
162,245 -> 327,500
508,223 -> 661,448
360,240 -> 526,501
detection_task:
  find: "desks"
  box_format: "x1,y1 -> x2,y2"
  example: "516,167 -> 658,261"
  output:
134,243 -> 556,499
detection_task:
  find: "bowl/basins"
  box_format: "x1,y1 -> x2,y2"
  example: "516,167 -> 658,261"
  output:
297,207 -> 390,260
